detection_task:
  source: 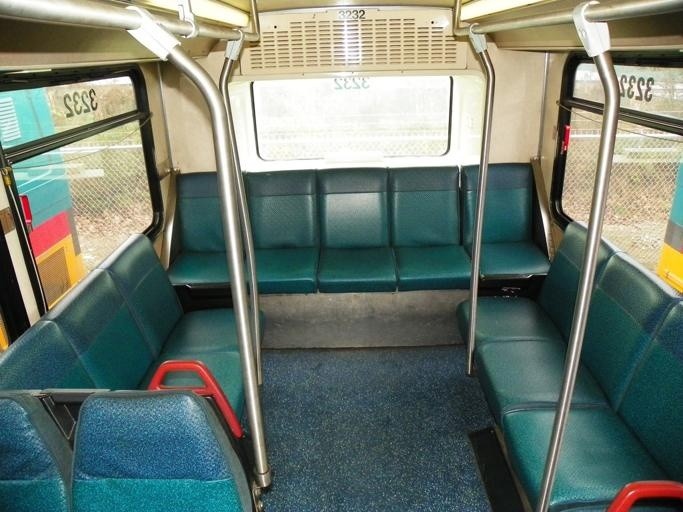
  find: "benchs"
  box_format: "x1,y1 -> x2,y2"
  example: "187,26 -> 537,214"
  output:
458,223 -> 682,510
1,233 -> 266,448
164,161 -> 556,309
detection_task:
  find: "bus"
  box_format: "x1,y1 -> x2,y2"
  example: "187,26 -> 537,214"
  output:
0,89 -> 87,347
655,157 -> 683,294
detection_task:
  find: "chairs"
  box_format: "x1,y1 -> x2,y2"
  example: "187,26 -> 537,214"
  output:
74,389 -> 253,510
2,392 -> 73,510
